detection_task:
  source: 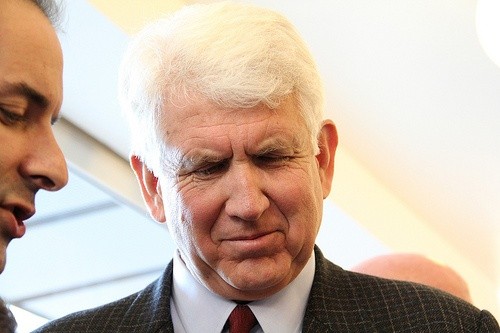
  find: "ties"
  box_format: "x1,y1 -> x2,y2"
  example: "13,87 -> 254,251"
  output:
228,305 -> 258,333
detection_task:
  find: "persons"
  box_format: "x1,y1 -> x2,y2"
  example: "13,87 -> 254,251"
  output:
0,0 -> 69,333
31,7 -> 500,333
